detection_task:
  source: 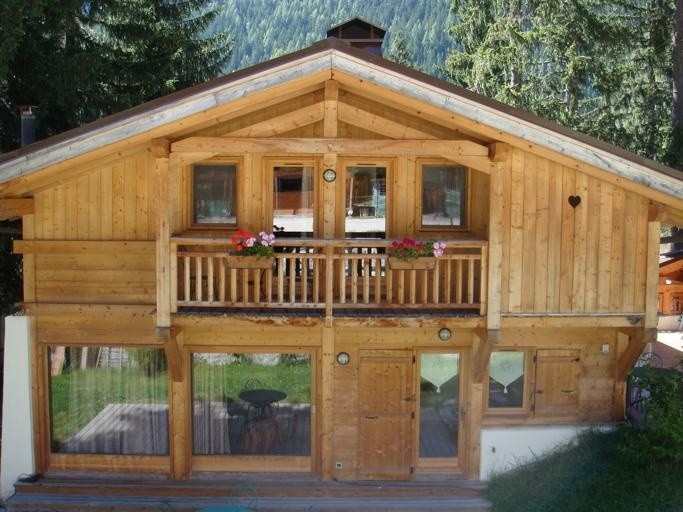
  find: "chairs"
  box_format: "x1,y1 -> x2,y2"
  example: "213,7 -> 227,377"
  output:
225,397 -> 249,429
245,378 -> 264,391
635,352 -> 663,409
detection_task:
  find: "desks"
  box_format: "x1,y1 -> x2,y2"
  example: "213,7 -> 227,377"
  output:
631,367 -> 681,408
239,389 -> 287,419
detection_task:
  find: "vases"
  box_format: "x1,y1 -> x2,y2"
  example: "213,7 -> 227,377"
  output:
388,256 -> 436,271
226,255 -> 276,269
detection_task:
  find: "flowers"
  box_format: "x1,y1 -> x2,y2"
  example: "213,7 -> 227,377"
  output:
390,237 -> 449,261
229,229 -> 276,258
